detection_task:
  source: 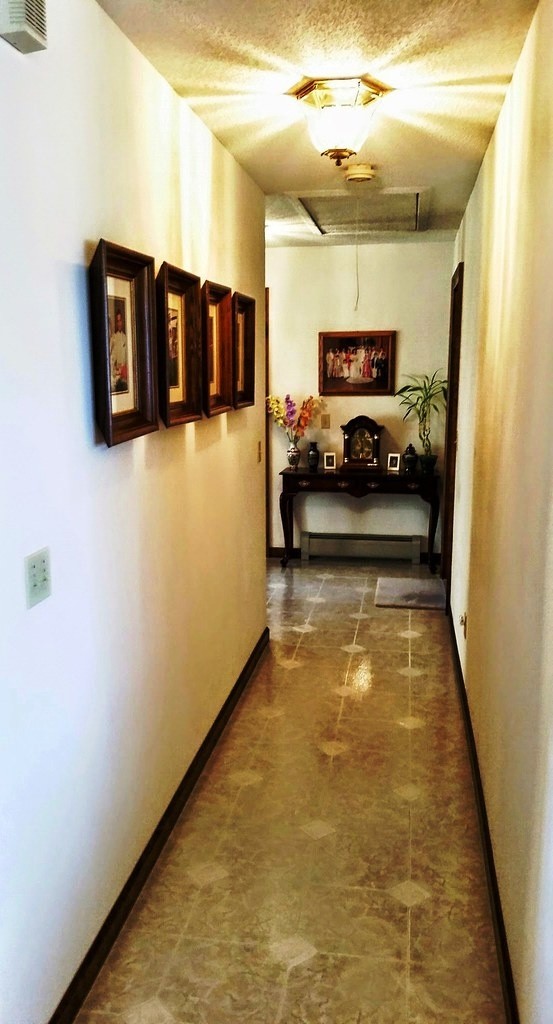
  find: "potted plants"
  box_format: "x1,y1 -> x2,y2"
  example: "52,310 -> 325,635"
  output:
394,366 -> 448,473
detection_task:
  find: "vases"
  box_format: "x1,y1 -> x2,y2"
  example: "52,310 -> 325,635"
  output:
308,442 -> 319,470
286,441 -> 301,469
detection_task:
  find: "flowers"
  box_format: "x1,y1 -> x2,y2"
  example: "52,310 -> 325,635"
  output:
267,392 -> 315,442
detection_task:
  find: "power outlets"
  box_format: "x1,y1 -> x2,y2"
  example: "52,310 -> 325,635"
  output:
26,547 -> 51,608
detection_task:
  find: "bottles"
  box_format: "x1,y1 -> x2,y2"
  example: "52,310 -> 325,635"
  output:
308,441 -> 319,473
402,444 -> 418,471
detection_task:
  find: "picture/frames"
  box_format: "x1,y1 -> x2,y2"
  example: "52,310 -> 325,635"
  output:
200,279 -> 236,418
324,452 -> 337,470
152,261 -> 204,427
387,453 -> 401,471
85,239 -> 159,448
317,330 -> 395,396
231,291 -> 255,409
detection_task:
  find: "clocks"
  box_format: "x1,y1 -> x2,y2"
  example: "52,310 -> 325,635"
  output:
340,415 -> 386,469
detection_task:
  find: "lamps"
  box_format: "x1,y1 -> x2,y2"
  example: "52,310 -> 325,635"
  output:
290,73 -> 391,168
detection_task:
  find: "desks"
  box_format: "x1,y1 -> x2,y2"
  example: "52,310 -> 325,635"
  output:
279,467 -> 439,575
